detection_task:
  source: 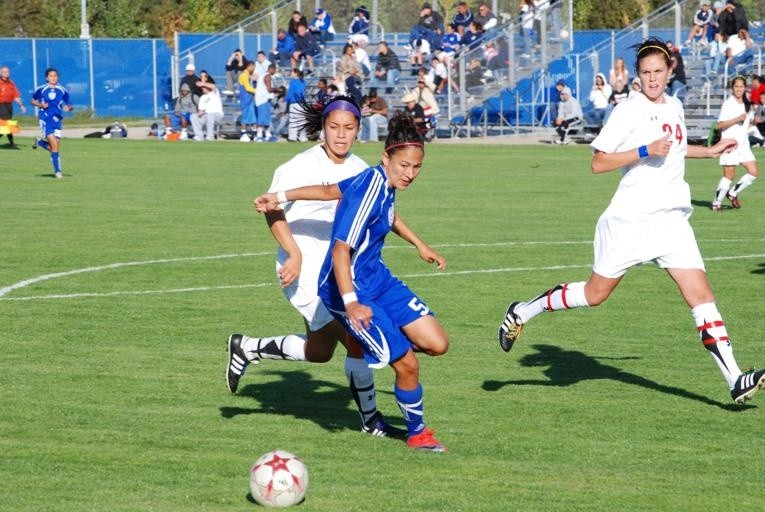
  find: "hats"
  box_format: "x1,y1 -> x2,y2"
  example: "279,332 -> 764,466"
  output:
401,94 -> 416,103
181,84 -> 191,91
315,9 -> 322,14
185,64 -> 194,71
421,3 -> 430,8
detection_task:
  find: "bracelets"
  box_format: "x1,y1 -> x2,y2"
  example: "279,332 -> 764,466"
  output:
342,292 -> 359,305
19,104 -> 24,107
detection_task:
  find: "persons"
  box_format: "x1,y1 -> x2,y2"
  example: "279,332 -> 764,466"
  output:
555,83 -> 582,143
404,1 -> 509,137
269,8 -> 336,79
712,75 -> 764,211
254,106 -> 450,451
227,92 -> 444,439
338,5 -> 402,142
686,0 -> 754,86
225,49 -> 339,142
664,41 -> 688,103
519,0 -> 563,57
30,67 -> 73,180
165,64 -> 224,141
500,38 -> 764,405
592,59 -> 641,127
0,67 -> 26,150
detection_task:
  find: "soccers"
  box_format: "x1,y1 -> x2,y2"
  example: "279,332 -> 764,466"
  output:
248,449 -> 308,507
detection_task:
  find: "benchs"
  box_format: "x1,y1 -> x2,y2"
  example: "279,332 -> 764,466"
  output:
172,35 -> 765,163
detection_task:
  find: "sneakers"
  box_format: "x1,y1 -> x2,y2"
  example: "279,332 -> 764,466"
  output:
226,334 -> 251,393
239,136 -> 278,142
731,366 -> 765,403
499,301 -> 524,352
712,192 -> 740,209
33,137 -> 62,179
162,133 -> 212,140
361,411 -> 448,452
